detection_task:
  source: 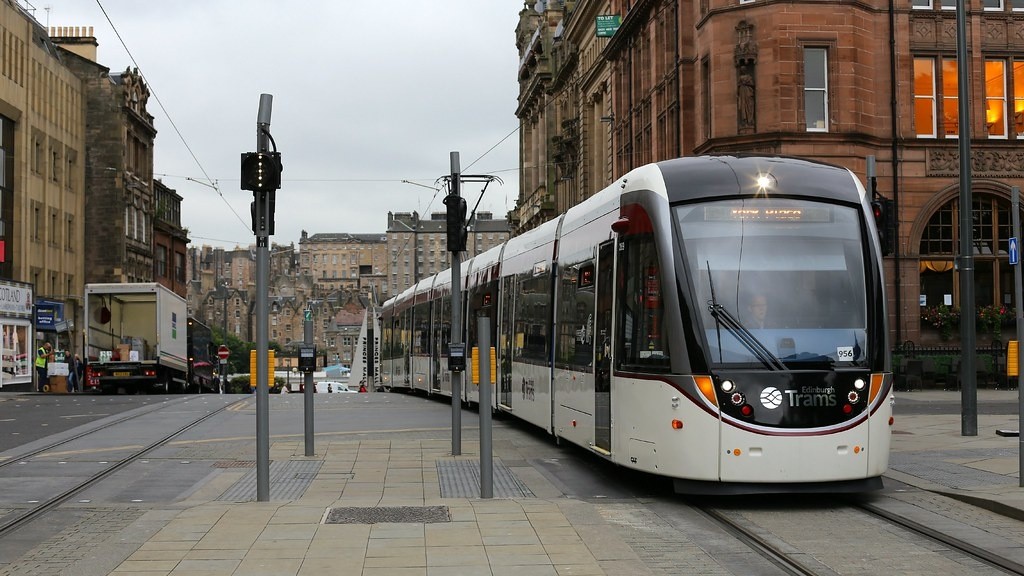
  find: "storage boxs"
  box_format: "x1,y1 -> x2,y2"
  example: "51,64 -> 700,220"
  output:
47,363 -> 69,378
99,336 -> 147,362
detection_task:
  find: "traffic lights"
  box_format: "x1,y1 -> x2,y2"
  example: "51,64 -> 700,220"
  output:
239,151 -> 283,191
444,193 -> 468,252
869,198 -> 898,258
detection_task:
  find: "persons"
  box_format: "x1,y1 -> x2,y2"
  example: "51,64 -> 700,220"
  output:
281,384 -> 289,394
328,384 -> 332,393
64,350 -> 82,392
36,343 -> 53,391
360,385 -> 366,392
272,383 -> 279,394
744,293 -> 778,328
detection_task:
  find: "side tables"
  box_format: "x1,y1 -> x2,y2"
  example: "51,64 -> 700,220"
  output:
0,314 -> 32,387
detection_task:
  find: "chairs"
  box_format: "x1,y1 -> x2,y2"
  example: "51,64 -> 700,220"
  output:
893,355 -> 1018,392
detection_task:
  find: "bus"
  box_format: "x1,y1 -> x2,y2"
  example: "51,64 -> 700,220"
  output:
376,155 -> 896,499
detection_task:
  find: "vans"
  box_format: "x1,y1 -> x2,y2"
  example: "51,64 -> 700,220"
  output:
316,381 -> 358,393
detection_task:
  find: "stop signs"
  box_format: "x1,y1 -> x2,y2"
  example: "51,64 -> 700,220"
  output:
217,345 -> 229,359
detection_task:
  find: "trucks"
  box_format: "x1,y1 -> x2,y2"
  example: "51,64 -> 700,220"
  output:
83,281 -> 189,394
186,314 -> 221,394
